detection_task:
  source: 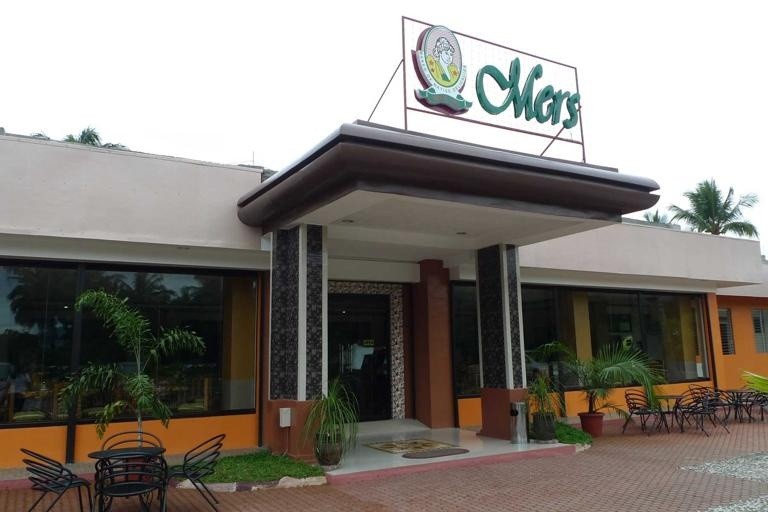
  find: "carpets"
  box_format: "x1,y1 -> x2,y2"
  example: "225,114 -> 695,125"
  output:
362,436 -> 460,454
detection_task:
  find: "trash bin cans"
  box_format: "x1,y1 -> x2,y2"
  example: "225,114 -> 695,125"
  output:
509,401 -> 527,444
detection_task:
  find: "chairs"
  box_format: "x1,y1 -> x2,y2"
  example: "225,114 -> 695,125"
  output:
612,384 -> 767,437
101,426 -> 170,511
12,444 -> 97,511
160,430 -> 227,512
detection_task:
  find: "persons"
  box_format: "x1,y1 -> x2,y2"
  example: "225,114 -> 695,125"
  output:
342,333 -> 387,418
6,360 -> 31,416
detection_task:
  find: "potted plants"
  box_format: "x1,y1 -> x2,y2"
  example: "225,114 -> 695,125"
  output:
302,375 -> 356,469
527,366 -> 557,444
531,338 -> 667,434
49,285 -> 213,486
